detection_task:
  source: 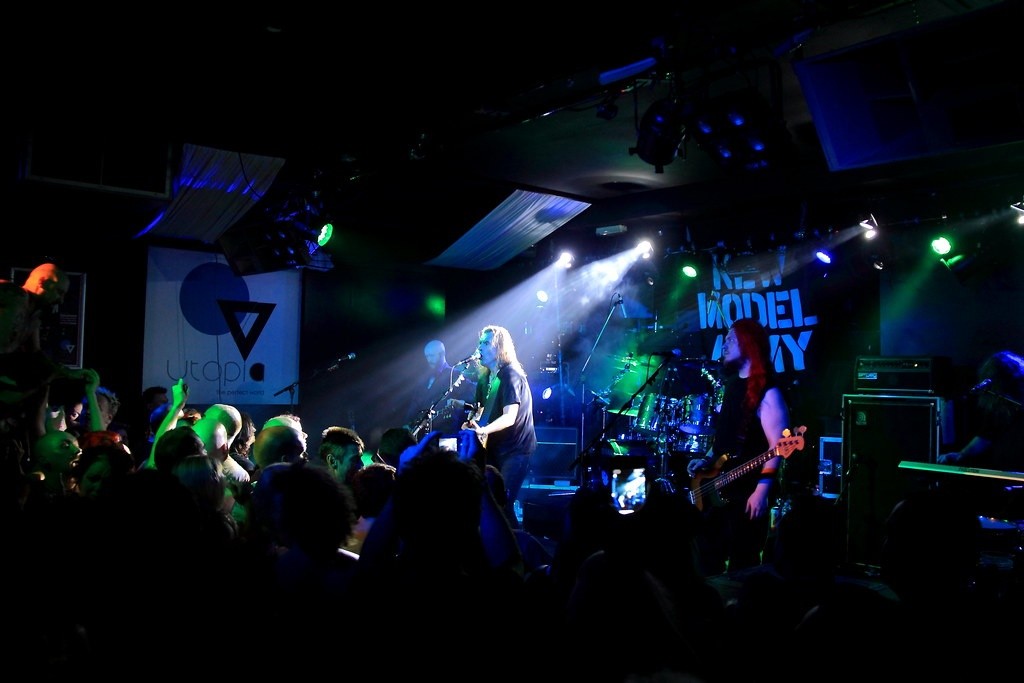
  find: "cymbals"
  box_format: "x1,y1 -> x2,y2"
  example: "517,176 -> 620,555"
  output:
681,357 -> 721,372
607,406 -> 640,417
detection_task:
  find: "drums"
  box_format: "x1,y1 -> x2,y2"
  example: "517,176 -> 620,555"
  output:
673,435 -> 708,453
677,394 -> 719,437
638,392 -> 677,437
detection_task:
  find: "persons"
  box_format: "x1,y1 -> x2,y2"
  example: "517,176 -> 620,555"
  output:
686,318 -> 790,581
2,263 -> 1023,682
403,341 -> 469,434
460,326 -> 537,529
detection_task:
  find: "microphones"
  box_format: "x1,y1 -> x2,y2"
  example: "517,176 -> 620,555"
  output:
655,312 -> 658,333
457,353 -> 481,365
707,290 -> 714,314
334,353 -> 356,365
972,378 -> 992,391
618,294 -> 628,319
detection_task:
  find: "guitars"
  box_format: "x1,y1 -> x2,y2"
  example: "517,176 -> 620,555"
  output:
466,417 -> 490,451
402,405 -> 456,442
687,425 -> 808,512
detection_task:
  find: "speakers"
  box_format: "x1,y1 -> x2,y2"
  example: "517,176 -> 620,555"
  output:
846,401 -> 933,559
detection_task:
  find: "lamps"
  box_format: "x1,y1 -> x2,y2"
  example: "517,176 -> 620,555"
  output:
874,255 -> 887,270
596,87 -> 767,174
812,228 -> 837,278
677,249 -> 706,284
261,191 -> 336,258
